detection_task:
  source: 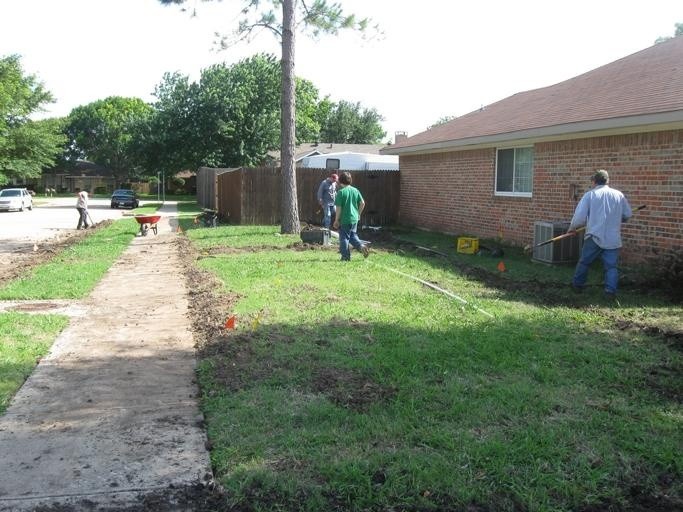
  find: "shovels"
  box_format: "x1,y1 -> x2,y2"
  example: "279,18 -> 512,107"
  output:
80,201 -> 96,228
413,246 -> 448,257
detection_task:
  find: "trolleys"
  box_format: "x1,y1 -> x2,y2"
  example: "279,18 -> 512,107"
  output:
134,213 -> 179,237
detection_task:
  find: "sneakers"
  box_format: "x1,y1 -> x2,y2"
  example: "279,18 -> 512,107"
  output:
571,281 -> 583,293
603,291 -> 617,302
340,257 -> 350,261
362,247 -> 369,258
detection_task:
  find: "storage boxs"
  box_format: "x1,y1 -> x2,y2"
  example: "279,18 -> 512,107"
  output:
457,237 -> 479,255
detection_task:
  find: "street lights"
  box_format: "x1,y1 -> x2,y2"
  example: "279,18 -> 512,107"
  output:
156,171 -> 161,201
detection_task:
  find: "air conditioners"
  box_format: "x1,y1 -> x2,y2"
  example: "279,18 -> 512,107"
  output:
531,220 -> 579,265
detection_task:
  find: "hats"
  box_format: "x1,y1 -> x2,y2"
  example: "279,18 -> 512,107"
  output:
588,170 -> 609,184
331,174 -> 339,181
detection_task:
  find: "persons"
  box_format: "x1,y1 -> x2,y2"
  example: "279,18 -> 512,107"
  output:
568,169 -> 632,301
75,189 -> 93,230
333,171 -> 368,261
317,174 -> 339,230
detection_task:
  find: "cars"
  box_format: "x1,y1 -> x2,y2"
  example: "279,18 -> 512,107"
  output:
110,189 -> 138,209
10,184 -> 35,197
0,187 -> 32,212
